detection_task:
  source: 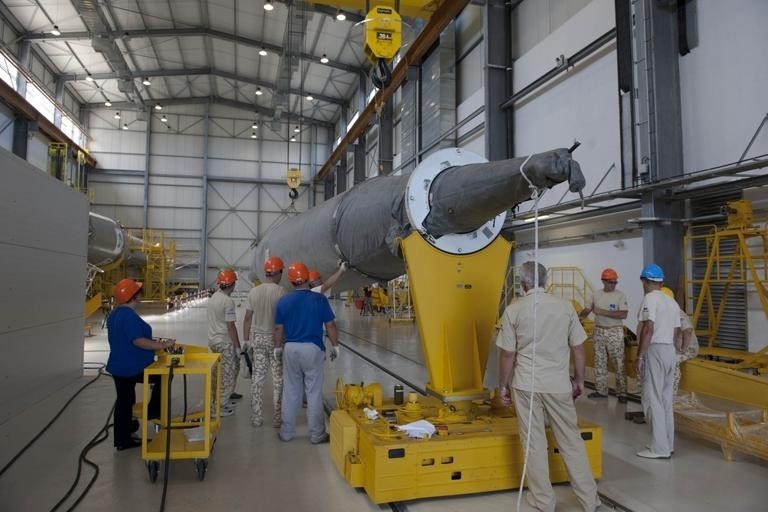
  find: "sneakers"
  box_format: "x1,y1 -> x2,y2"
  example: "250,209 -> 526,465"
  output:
209,408 -> 233,417
226,401 -> 238,408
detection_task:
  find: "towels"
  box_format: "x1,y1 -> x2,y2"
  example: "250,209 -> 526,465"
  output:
360,405 -> 380,422
183,423 -> 209,442
400,415 -> 438,438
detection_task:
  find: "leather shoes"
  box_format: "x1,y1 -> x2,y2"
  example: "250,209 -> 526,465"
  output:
588,391 -> 607,399
230,391 -> 244,399
118,441 -> 143,450
619,396 -> 627,404
647,443 -> 675,455
524,492 -> 538,507
636,450 -> 672,459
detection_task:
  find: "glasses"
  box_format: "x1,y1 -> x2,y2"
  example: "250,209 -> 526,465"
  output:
603,279 -> 620,285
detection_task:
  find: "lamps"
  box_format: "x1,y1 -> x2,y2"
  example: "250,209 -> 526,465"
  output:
51,25 -> 60,36
114,112 -> 120,119
122,123 -> 128,129
264,3 -> 274,11
306,92 -> 311,100
259,47 -> 267,56
105,99 -> 111,106
256,87 -> 263,95
143,77 -> 151,85
86,74 -> 93,82
155,102 -> 162,109
251,132 -> 256,139
320,54 -> 329,63
294,126 -> 300,133
252,122 -> 258,129
290,135 -> 296,142
337,13 -> 346,21
161,115 -> 167,121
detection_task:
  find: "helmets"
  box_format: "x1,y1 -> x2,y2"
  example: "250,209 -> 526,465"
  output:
288,262 -> 310,284
263,255 -> 286,278
114,278 -> 142,302
601,267 -> 618,280
309,271 -> 318,285
215,267 -> 239,290
641,263 -> 667,283
661,286 -> 676,300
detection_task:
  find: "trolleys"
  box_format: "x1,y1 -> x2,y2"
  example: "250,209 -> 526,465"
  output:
132,342 -> 223,483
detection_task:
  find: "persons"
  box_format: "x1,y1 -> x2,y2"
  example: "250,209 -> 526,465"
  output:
274,262 -> 339,444
242,256 -> 285,427
495,260 -> 603,512
309,262 -> 348,344
579,269 -> 630,403
206,268 -> 242,419
105,278 -> 176,450
660,285 -> 700,395
634,263 -> 681,460
364,286 -> 376,315
230,391 -> 242,399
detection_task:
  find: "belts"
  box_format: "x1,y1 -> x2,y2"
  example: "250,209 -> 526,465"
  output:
595,324 -> 616,329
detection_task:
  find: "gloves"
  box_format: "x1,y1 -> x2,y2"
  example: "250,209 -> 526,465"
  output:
235,348 -> 243,360
329,345 -> 340,362
273,347 -> 284,364
243,342 -> 252,353
